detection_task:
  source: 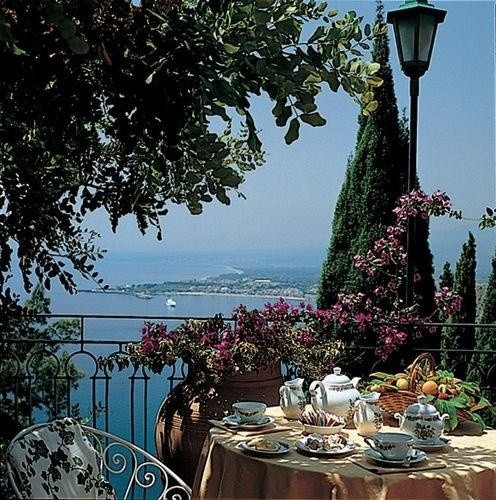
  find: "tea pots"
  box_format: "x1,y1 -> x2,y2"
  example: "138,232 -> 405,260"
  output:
353,390 -> 383,436
392,395 -> 450,443
309,366 -> 362,419
278,378 -> 306,418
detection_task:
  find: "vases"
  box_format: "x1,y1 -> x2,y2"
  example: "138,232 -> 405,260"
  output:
154,360 -> 284,500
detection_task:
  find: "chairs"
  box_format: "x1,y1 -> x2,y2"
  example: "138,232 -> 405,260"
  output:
1,416 -> 192,500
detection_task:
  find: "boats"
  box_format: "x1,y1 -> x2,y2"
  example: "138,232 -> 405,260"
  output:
135,290 -> 153,299
163,298 -> 176,307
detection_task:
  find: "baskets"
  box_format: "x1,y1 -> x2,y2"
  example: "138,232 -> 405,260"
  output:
365,352 -> 474,424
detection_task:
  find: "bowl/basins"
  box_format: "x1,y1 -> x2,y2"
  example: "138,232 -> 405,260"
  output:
302,423 -> 346,435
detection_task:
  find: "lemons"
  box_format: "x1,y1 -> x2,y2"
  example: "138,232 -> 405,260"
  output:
395,378 -> 409,390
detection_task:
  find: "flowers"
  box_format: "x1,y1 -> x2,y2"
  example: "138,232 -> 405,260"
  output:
126,189 -> 453,379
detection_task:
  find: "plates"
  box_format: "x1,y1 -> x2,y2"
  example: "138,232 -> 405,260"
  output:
224,415 -> 273,430
413,438 -> 453,452
363,447 -> 426,463
295,437 -> 357,454
244,439 -> 293,454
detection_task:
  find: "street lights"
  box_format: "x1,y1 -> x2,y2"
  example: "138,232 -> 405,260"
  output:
385,0 -> 446,310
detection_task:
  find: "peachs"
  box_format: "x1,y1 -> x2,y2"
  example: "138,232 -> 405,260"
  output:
422,381 -> 439,396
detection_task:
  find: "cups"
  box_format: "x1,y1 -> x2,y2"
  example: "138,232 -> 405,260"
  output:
232,402 -> 267,422
363,432 -> 415,460
